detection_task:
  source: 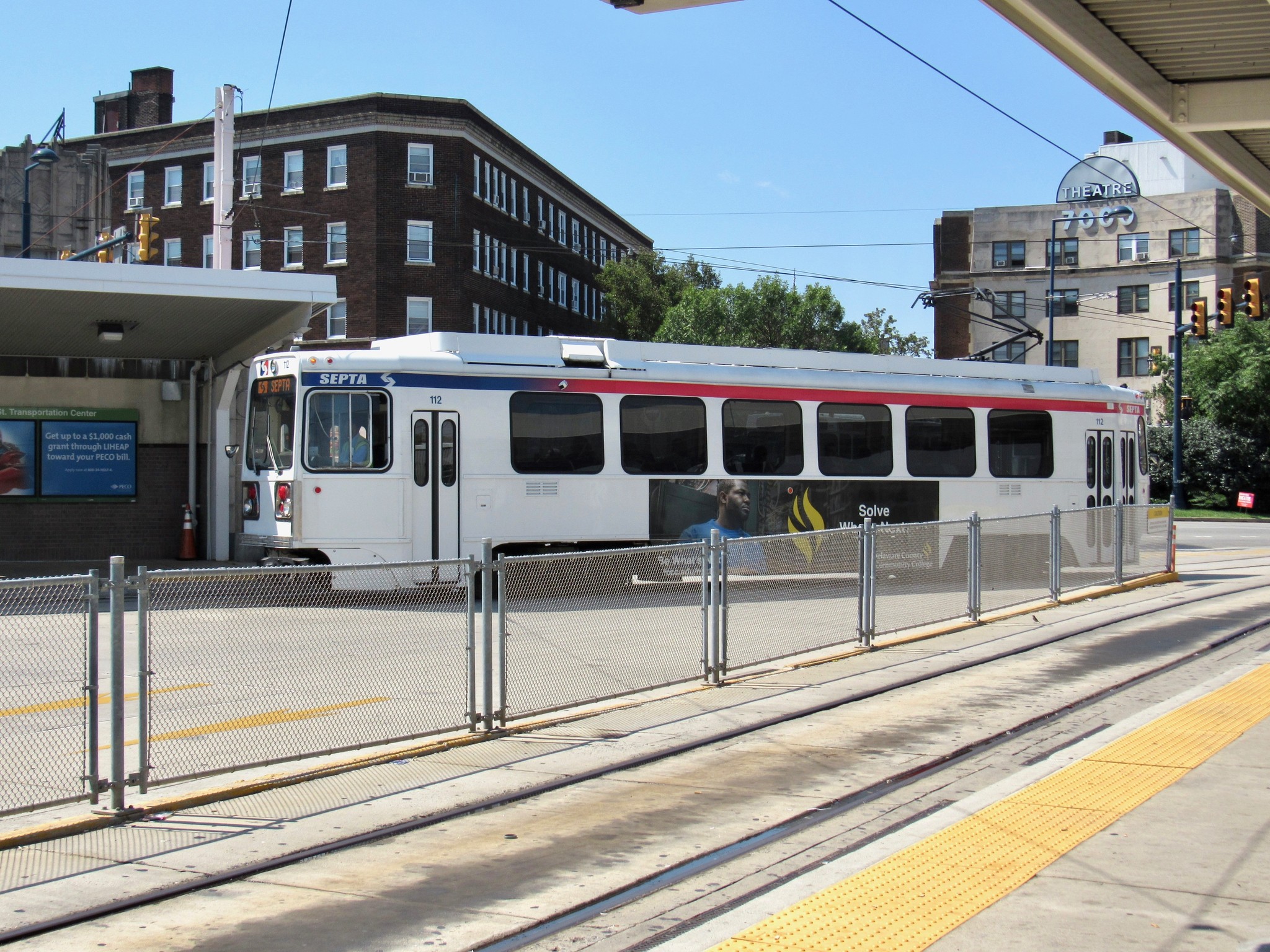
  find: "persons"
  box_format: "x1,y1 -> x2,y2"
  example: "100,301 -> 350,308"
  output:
331,411 -> 373,468
668,479 -> 767,575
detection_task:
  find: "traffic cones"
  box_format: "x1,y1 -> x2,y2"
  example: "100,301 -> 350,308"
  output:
180,504 -> 197,560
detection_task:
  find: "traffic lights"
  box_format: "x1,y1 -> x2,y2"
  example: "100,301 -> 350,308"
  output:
1216,288 -> 1232,325
137,213 -> 161,261
60,250 -> 76,261
1189,301 -> 1206,336
97,231 -> 115,263
1243,277 -> 1261,319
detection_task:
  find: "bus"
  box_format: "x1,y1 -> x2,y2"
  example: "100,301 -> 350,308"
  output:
234,287 -> 1151,610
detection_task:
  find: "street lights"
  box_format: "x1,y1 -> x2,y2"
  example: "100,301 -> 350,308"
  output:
21,149 -> 61,259
1048,206 -> 1133,367
